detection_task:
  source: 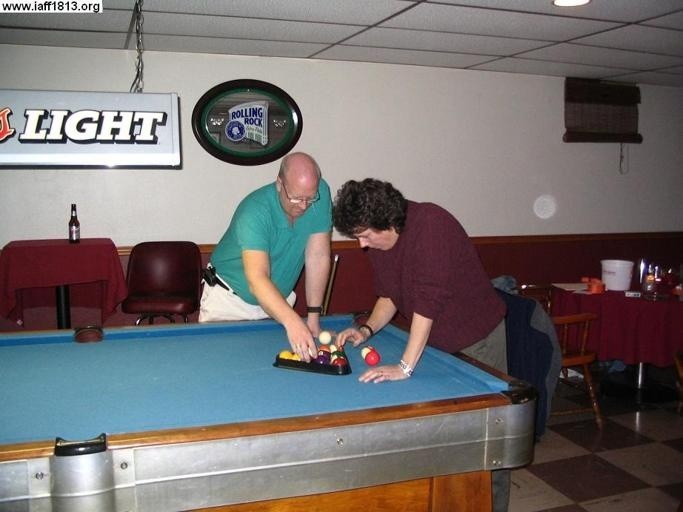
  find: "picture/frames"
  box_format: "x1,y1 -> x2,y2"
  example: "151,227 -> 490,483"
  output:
209,129 -> 222,145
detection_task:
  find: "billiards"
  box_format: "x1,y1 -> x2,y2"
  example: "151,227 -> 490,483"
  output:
319,331 -> 331,345
279,344 -> 346,366
361,345 -> 380,366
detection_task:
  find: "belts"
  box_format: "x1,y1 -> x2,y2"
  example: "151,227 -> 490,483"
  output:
214,274 -> 238,296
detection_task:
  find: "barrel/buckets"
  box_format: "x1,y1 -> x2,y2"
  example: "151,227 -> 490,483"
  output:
600,257 -> 635,292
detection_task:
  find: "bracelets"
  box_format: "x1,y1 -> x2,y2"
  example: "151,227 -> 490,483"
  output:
358,325 -> 374,337
398,359 -> 413,377
306,305 -> 324,316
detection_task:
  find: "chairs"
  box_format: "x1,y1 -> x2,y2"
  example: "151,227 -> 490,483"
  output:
510,283 -> 552,313
122,240 -> 205,326
293,253 -> 339,316
546,311 -> 603,429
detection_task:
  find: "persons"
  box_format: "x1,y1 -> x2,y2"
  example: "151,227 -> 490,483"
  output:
331,179 -> 508,380
198,153 -> 334,364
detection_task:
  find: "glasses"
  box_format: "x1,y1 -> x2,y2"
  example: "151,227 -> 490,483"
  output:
282,183 -> 321,204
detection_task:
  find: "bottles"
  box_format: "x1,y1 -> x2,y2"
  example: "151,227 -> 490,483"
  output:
68,204 -> 80,244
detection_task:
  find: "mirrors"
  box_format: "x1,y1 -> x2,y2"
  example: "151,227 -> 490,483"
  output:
190,76 -> 303,166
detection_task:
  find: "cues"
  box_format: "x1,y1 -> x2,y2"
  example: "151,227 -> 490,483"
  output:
323,255 -> 338,315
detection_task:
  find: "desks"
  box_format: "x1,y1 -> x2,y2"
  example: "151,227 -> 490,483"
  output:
0,308 -> 539,512
540,274 -> 683,388
0,238 -> 127,328
